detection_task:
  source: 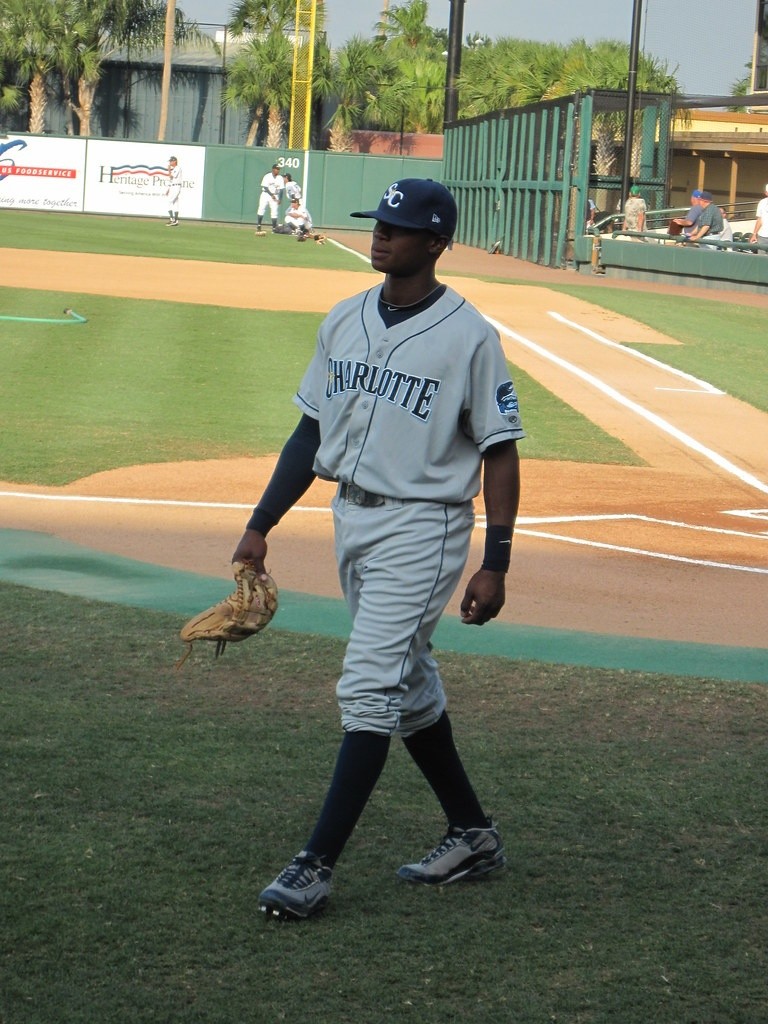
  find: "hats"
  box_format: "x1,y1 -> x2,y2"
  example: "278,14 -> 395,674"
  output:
763,182 -> 768,192
272,164 -> 280,169
348,178 -> 460,242
282,173 -> 290,177
691,190 -> 703,200
168,156 -> 177,164
629,184 -> 643,197
290,198 -> 300,203
700,191 -> 713,201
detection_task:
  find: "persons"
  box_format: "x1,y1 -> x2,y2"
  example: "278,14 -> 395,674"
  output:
166,156 -> 183,226
672,190 -> 733,252
585,199 -> 595,235
622,185 -> 650,243
257,164 -> 312,241
750,184 -> 768,254
232,178 -> 525,922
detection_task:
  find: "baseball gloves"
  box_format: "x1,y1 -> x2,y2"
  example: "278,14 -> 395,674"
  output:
181,556 -> 282,646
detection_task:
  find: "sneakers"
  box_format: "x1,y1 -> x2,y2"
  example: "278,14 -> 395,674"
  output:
255,851 -> 337,921
396,826 -> 510,883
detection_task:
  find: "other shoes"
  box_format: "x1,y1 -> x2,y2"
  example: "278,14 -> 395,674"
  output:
165,219 -> 178,227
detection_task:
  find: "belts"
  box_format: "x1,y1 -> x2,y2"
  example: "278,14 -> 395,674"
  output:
337,481 -> 385,508
